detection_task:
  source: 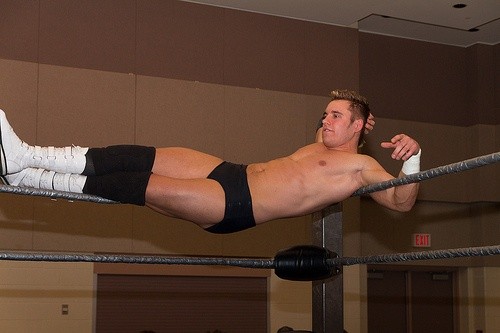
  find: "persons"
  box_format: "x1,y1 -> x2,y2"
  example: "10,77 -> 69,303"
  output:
0,89 -> 422,234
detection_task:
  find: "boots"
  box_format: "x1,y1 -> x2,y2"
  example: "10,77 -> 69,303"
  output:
0,167 -> 87,194
0,109 -> 90,177
